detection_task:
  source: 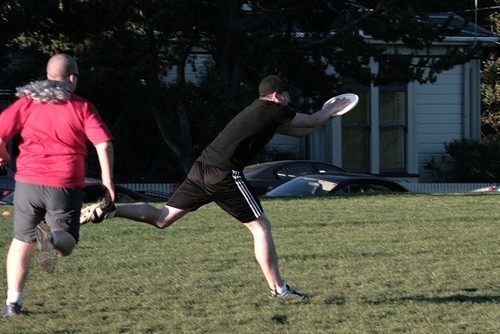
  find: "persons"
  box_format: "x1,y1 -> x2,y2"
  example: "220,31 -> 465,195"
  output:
0,54 -> 115,317
79,76 -> 352,300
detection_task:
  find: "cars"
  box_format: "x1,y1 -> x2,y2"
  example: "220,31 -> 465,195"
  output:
3,176 -> 156,203
243,159 -> 349,195
265,172 -> 410,197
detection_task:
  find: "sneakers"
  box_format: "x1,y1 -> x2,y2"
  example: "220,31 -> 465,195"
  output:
35,222 -> 57,273
268,283 -> 308,299
79,201 -> 118,225
0,302 -> 22,317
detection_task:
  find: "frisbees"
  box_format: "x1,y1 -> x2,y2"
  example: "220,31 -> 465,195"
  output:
322,92 -> 359,117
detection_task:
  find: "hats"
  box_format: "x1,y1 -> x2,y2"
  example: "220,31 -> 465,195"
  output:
258,75 -> 290,96
